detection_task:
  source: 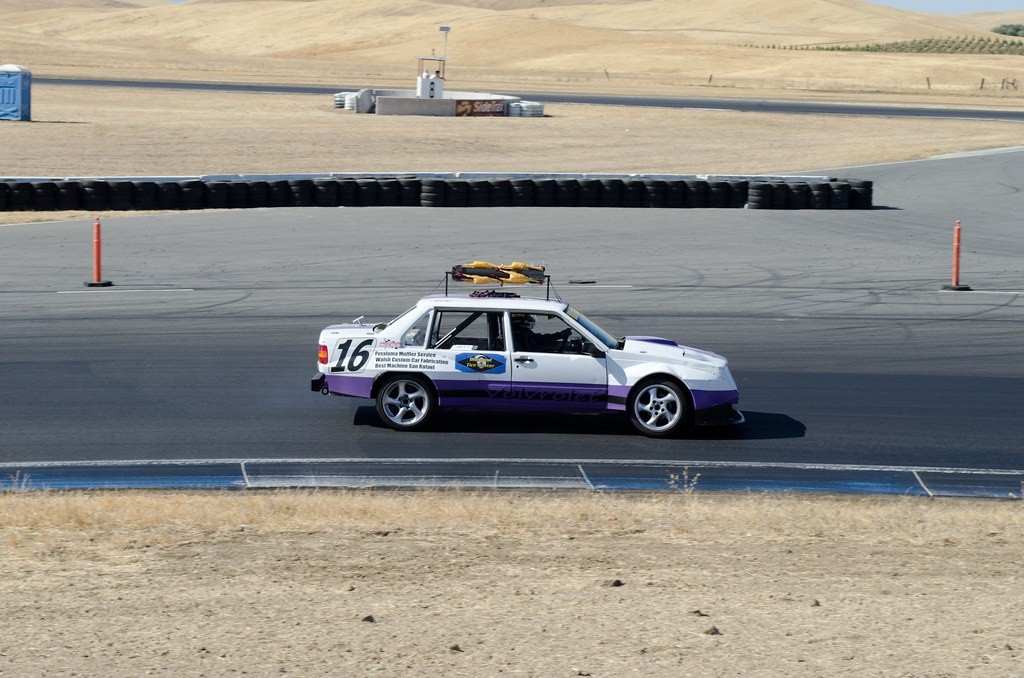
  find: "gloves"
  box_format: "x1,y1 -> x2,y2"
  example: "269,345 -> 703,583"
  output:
555,328 -> 572,339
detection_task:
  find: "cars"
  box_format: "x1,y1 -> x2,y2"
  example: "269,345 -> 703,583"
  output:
310,294 -> 744,438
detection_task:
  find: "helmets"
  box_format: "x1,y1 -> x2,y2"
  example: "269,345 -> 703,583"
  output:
521,314 -> 537,329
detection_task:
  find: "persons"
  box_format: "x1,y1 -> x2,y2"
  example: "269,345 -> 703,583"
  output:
513,315 -> 572,352
432,71 -> 443,79
